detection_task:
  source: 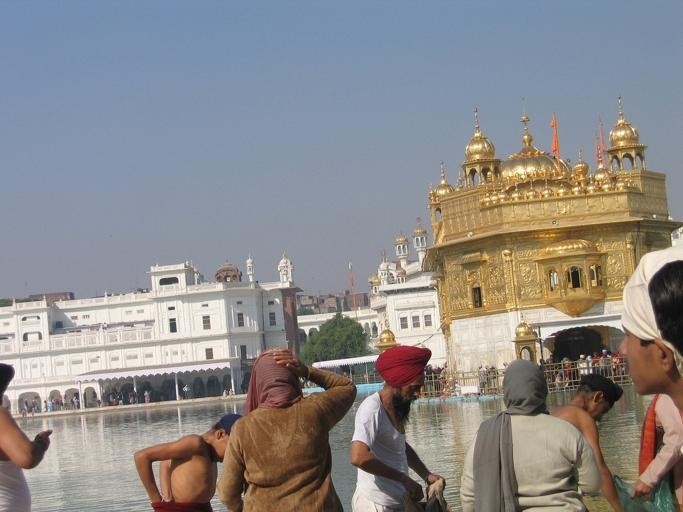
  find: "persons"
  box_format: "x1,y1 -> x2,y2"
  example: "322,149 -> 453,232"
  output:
133,413 -> 244,512
350,344 -> 447,511
298,366 -> 383,388
458,358 -> 603,512
0,362 -> 52,512
17,382 -> 191,418
421,361 -> 458,398
630,393 -> 682,511
215,347 -> 357,511
549,372 -> 624,511
476,347 -> 626,395
616,243 -> 683,413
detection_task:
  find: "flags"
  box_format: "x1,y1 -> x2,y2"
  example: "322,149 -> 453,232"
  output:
599,121 -> 606,166
594,136 -> 600,165
552,115 -> 560,158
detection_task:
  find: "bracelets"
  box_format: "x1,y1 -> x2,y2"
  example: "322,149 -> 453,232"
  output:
421,470 -> 429,484
